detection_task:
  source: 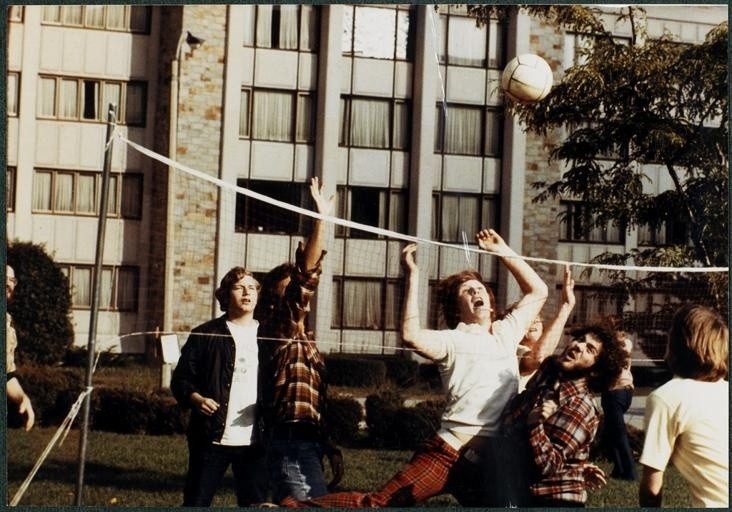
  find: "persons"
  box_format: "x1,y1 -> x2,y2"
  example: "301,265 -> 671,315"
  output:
170,266 -> 264,507
517,262 -> 578,394
253,174 -> 337,507
4,263 -> 36,433
593,311 -> 640,481
282,230 -> 551,508
639,303 -> 731,510
501,320 -> 630,511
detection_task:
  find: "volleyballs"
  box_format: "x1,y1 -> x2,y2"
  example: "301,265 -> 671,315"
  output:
502,53 -> 553,105
